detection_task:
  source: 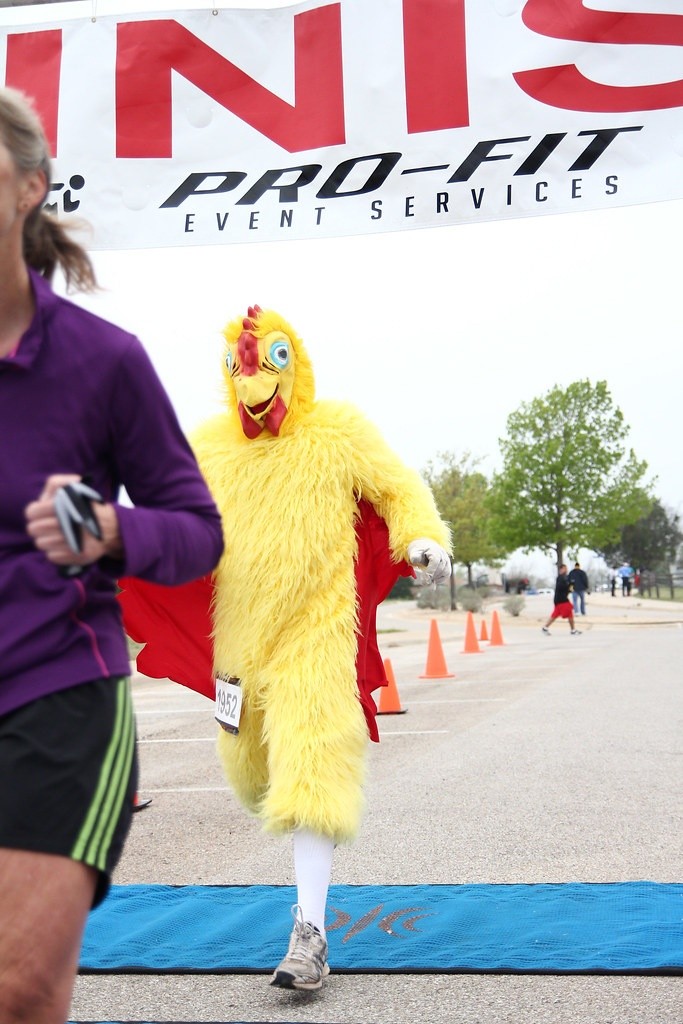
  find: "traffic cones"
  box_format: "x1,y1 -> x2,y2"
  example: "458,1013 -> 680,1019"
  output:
415,617 -> 456,679
486,611 -> 509,647
460,610 -> 484,656
375,657 -> 410,715
476,619 -> 492,643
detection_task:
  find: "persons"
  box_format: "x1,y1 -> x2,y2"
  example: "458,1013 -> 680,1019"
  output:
542,564 -> 584,635
608,565 -> 617,596
0,88 -> 225,1024
568,562 -> 590,617
617,562 -> 636,597
115,305 -> 457,990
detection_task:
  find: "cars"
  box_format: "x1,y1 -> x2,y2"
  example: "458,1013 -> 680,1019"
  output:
526,586 -> 555,596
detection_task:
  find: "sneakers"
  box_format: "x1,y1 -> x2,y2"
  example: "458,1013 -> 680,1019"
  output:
270,904 -> 330,990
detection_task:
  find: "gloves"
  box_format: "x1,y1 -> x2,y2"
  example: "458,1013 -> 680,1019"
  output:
406,538 -> 451,584
55,481 -> 106,580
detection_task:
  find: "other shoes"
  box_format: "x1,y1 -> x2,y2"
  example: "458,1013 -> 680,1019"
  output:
571,630 -> 582,635
540,625 -> 551,636
133,793 -> 153,811
